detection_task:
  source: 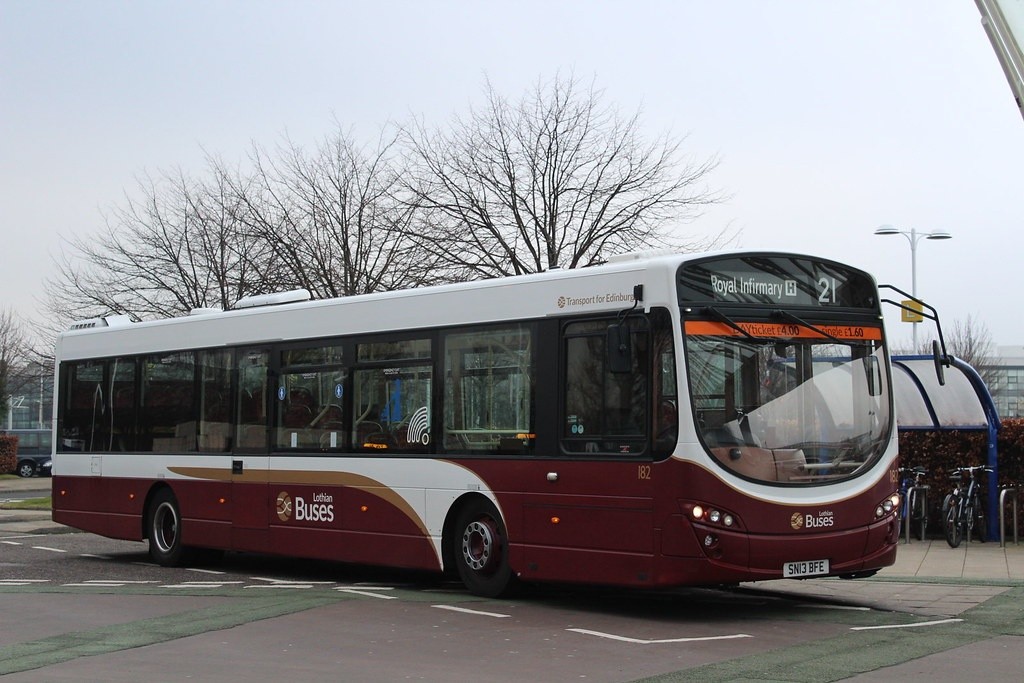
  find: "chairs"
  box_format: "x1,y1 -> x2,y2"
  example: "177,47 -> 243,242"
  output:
281,428 -> 314,448
251,387 -> 262,425
322,421 -> 342,448
241,423 -> 266,449
217,387 -> 257,424
357,422 -> 384,447
318,404 -> 342,428
387,421 -> 410,448
284,404 -> 315,428
290,386 -> 316,412
206,422 -> 232,450
364,433 -> 398,448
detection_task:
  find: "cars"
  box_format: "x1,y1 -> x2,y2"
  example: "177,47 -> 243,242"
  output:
36,456 -> 53,476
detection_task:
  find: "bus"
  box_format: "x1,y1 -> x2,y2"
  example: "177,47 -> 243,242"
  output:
51,248 -> 959,597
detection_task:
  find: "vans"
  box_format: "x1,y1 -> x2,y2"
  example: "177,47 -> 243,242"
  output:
0,427 -> 53,478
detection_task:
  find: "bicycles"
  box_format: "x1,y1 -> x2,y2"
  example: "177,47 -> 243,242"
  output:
896,466 -> 944,541
941,465 -> 997,549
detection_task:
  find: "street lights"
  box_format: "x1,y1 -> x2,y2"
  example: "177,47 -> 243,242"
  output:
874,227 -> 953,356
